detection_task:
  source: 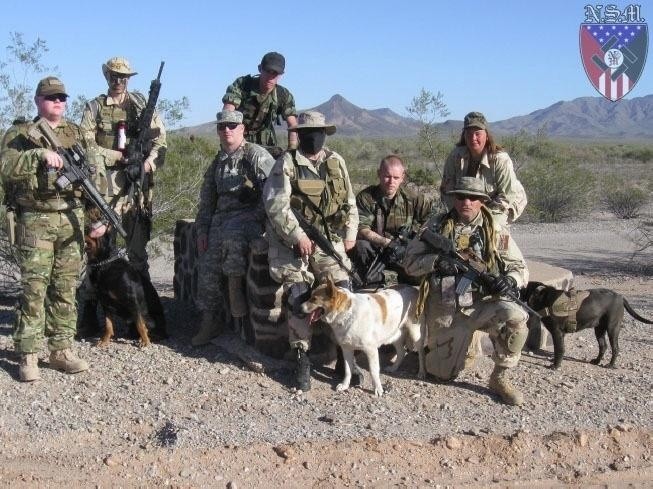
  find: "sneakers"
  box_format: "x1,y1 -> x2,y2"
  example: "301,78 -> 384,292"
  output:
48,349 -> 89,374
192,308 -> 222,346
18,351 -> 39,381
488,365 -> 524,406
291,352 -> 311,391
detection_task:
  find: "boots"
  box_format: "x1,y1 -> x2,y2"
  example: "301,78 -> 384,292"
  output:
77,300 -> 99,338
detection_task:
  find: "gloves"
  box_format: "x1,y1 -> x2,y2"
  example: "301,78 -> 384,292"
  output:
387,241 -> 407,263
435,255 -> 469,276
491,276 -> 516,296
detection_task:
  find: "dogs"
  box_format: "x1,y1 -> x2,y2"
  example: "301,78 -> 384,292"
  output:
79,220 -> 168,350
519,279 -> 653,371
299,270 -> 429,398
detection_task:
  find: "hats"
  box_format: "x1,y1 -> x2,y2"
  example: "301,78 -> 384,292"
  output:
100,56 -> 138,78
36,76 -> 69,98
445,176 -> 491,202
213,110 -> 245,125
463,112 -> 487,131
287,111 -> 336,136
261,52 -> 285,74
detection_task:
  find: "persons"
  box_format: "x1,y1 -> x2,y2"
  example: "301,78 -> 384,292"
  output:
222,52 -> 298,158
190,109 -> 276,346
1,78 -> 89,382
76,56 -> 168,338
356,156 -> 438,286
263,110 -> 365,391
441,112 -> 517,227
404,176 -> 530,405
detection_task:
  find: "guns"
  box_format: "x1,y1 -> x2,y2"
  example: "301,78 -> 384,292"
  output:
291,207 -> 362,285
124,61 -> 164,202
420,228 -> 544,320
365,224 -> 414,286
37,120 -> 128,237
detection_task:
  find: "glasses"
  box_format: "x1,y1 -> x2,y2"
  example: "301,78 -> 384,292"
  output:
454,194 -> 481,201
215,124 -> 239,130
42,95 -> 67,102
